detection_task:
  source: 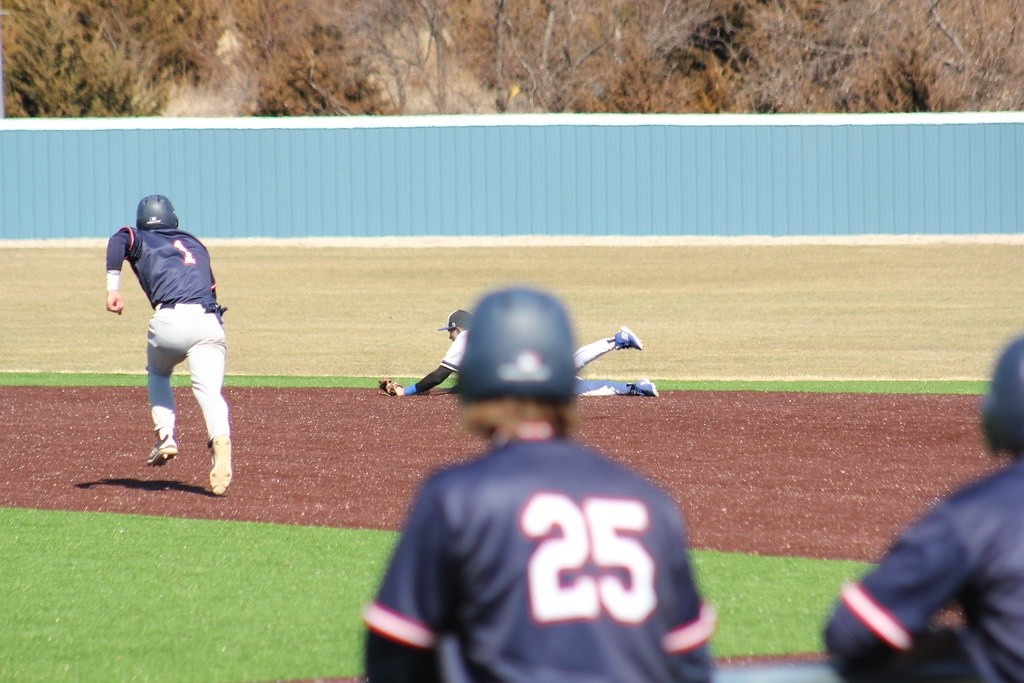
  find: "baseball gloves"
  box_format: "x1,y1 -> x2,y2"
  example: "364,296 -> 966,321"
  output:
378,379 -> 403,396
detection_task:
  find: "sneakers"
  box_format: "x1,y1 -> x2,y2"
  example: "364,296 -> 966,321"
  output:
627,379 -> 659,397
614,326 -> 645,350
209,437 -> 233,495
147,439 -> 179,466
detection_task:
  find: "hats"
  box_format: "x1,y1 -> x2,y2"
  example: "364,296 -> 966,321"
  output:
438,310 -> 474,330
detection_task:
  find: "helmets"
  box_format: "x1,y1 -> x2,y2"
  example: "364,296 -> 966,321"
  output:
981,336 -> 1023,452
458,288 -> 573,406
136,195 -> 178,229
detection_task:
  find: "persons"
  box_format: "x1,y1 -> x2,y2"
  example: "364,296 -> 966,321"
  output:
103,195 -> 234,495
823,332 -> 1024,683
358,287 -> 714,683
378,309 -> 661,398
